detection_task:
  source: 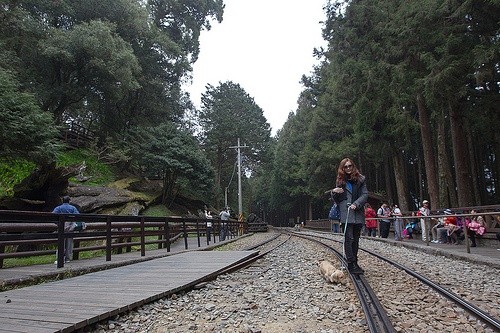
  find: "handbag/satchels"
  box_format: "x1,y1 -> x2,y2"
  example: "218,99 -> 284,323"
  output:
446,223 -> 460,236
327,189 -> 340,220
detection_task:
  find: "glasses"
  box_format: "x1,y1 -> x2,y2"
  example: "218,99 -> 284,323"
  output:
344,165 -> 353,168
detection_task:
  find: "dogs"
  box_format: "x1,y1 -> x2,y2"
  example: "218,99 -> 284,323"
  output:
319,260 -> 347,285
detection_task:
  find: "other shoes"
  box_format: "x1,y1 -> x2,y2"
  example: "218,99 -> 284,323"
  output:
430,239 -> 460,245
471,244 -> 477,247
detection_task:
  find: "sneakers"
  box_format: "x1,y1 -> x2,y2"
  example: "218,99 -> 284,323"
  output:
347,262 -> 364,273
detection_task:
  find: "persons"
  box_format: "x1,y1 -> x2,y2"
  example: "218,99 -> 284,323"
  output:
419,200 -> 430,243
247,212 -> 257,233
333,202 -> 500,251
377,201 -> 390,238
205,210 -> 213,241
364,203 -> 376,237
219,208 -> 230,240
332,157 -> 369,275
52,196 -> 81,264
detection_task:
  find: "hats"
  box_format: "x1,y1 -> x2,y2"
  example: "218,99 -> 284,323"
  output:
422,200 -> 429,203
444,209 -> 452,215
